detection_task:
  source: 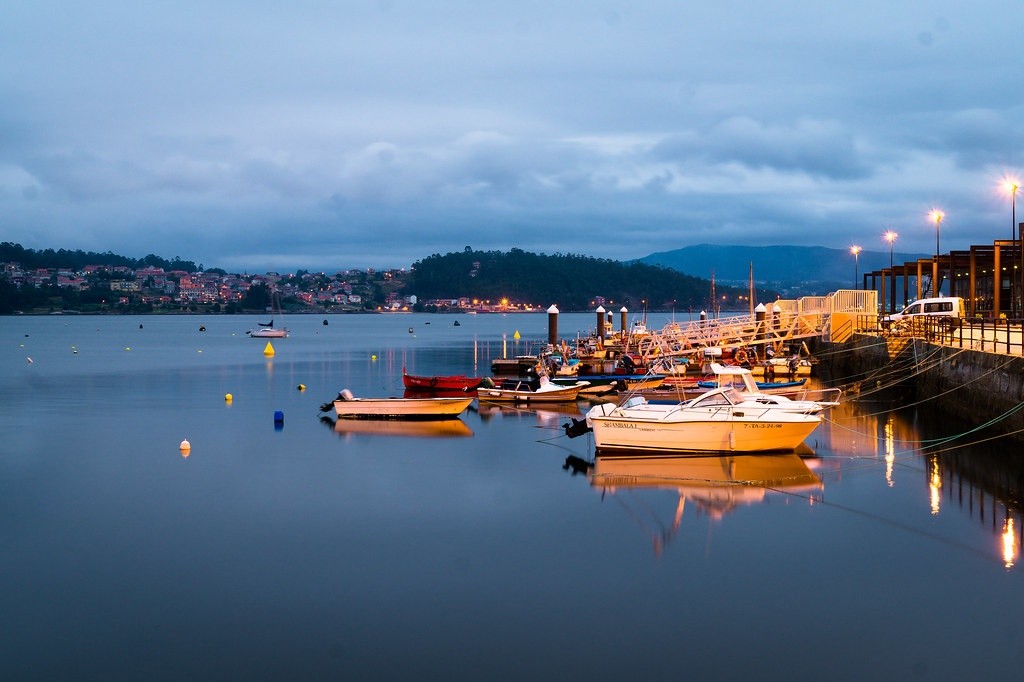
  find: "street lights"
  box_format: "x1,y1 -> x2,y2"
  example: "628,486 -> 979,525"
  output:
928,209 -> 945,298
1003,174 -> 1019,327
882,229 -> 898,315
850,245 -> 862,311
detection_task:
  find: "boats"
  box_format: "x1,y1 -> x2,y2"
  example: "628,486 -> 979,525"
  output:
562,356 -> 823,452
563,452 -> 825,534
317,416 -> 473,437
402,366 -> 507,392
478,401 -> 581,417
251,326 -> 286,338
490,336 -> 842,415
476,363 -> 584,401
317,388 -> 474,416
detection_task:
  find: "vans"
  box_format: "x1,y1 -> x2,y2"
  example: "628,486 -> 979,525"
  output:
880,296 -> 966,333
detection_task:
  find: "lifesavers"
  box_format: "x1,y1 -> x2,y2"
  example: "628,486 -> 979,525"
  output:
735,351 -> 748,363
430,378 -> 438,387
622,337 -> 628,347
